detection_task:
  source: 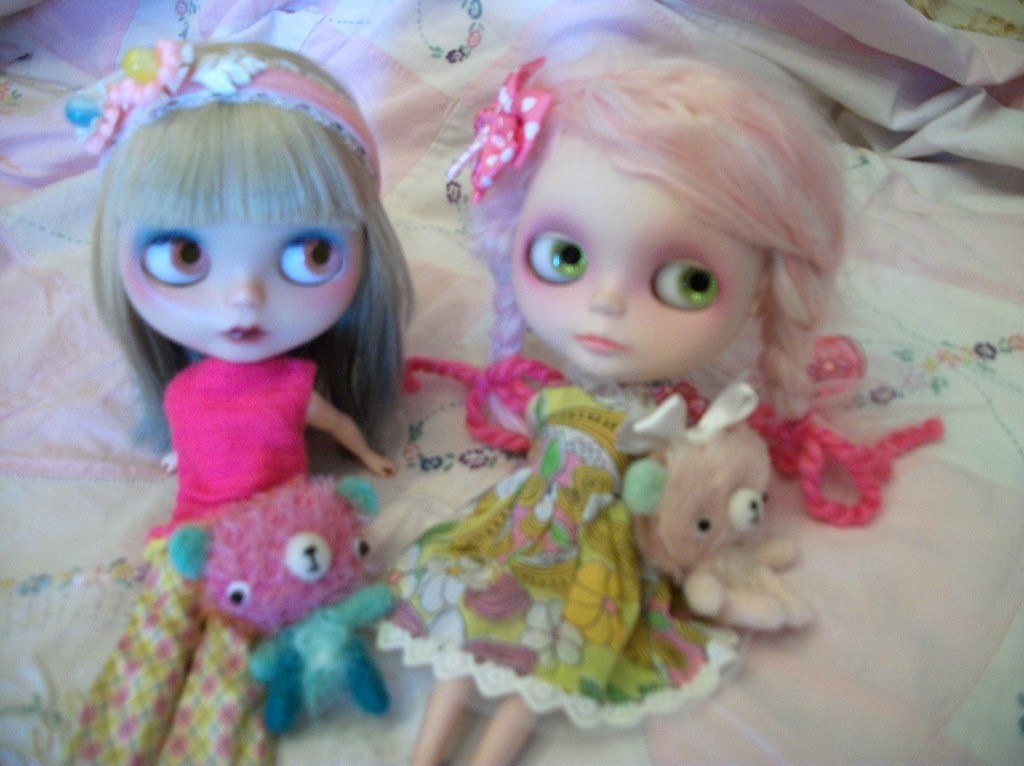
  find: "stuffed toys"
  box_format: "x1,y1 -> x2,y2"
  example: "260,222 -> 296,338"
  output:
167,474 -> 400,737
622,383 -> 816,636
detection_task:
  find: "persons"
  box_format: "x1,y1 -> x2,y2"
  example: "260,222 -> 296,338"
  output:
369,57 -> 849,765
62,45 -> 412,766
